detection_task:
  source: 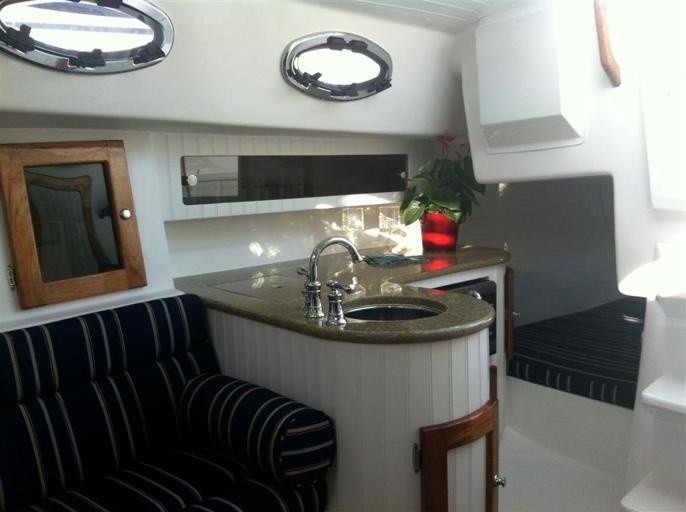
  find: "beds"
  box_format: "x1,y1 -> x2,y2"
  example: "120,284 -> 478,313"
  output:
501,296 -> 648,410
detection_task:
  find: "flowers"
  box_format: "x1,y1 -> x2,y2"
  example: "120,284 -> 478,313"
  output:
398,134 -> 486,222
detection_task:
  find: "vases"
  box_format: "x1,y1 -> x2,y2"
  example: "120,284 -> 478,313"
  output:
422,207 -> 458,252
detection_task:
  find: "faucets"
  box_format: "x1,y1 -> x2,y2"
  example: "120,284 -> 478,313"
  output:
307,237 -> 363,319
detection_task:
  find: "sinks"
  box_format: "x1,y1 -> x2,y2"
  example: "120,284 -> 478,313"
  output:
342,296 -> 448,324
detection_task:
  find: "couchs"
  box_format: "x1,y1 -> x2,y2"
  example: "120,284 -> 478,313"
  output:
0,294 -> 336,512
